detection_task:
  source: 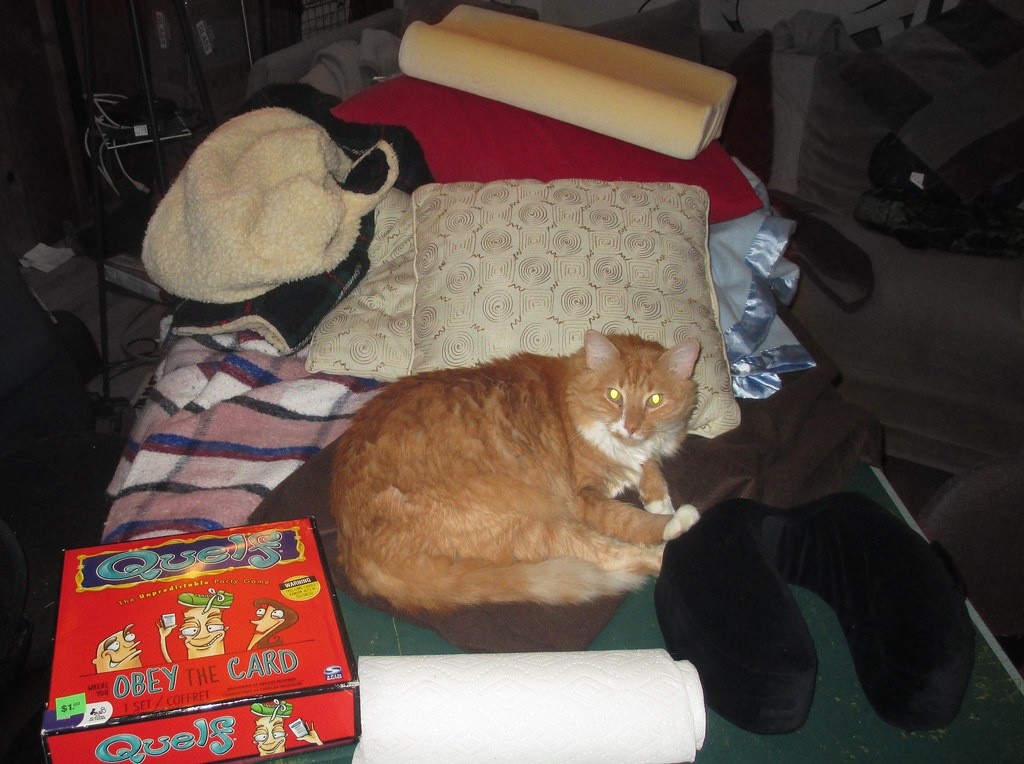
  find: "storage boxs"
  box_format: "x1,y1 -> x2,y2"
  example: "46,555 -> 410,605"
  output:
41,515 -> 361,764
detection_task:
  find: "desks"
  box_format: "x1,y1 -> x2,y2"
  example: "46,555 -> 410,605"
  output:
248,463 -> 1024,764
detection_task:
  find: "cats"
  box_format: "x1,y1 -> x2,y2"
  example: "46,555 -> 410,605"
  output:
329,326 -> 702,616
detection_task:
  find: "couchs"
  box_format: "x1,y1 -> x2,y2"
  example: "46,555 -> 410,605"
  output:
796,0 -> 1024,420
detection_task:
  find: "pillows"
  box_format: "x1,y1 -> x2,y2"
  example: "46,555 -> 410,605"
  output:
305,188 -> 414,383
409,180 -> 743,439
